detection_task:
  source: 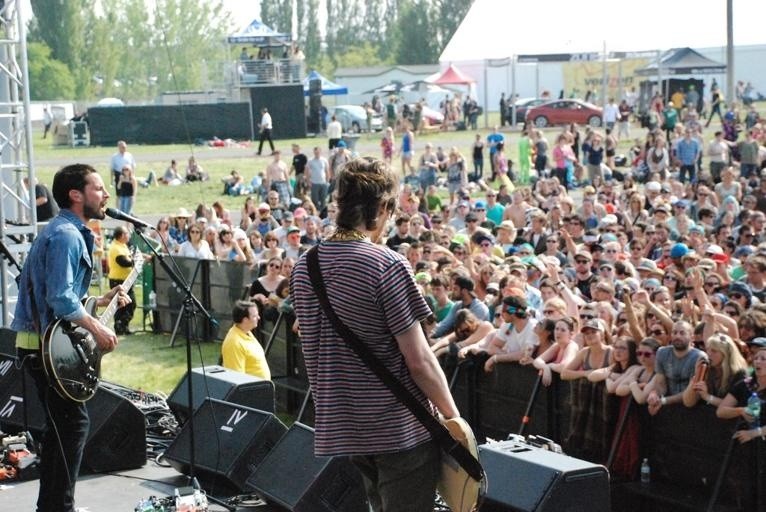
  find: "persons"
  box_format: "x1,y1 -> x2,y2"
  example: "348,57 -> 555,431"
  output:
118,167 -> 135,217
107,226 -> 135,336
253,108 -> 279,157
40,108 -> 90,145
221,302 -> 272,382
137,135 -> 300,336
288,156 -> 461,511
233,43 -> 306,83
9,163 -> 134,511
254,75 -> 766,445
24,176 -> 59,222
110,140 -> 136,198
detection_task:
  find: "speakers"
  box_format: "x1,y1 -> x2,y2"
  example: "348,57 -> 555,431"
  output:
244,421 -> 365,511
0,327 -> 46,442
475,433 -> 611,511
38,385 -> 146,477
165,365 -> 278,412
162,396 -> 289,495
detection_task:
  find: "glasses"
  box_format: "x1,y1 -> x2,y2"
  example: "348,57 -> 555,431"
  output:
726,312 -> 738,316
664,275 -> 677,281
468,220 -> 477,224
578,304 -> 593,310
742,200 -> 752,204
647,313 -> 659,319
269,263 -> 280,269
705,254 -> 711,257
711,302 -> 723,308
290,235 -> 300,239
487,194 -> 495,197
646,232 -> 655,235
605,229 -> 615,233
577,259 -> 589,264
480,244 -> 489,247
270,197 -> 277,201
266,239 -> 276,242
439,241 -> 448,245
697,192 -> 708,196
713,330 -> 730,352
636,350 -> 653,358
649,329 -> 666,335
569,222 -> 579,226
645,286 -> 655,291
476,209 -> 484,212
432,221 -> 441,224
586,192 -> 594,196
739,324 -> 755,330
580,314 -> 593,319
190,230 -> 200,234
446,210 -> 450,214
671,310 -> 682,314
705,281 -> 719,288
221,231 -> 230,236
177,217 -> 186,219
743,271 -> 760,274
728,293 -> 745,299
607,250 -> 617,253
542,309 -> 558,315
676,205 -> 685,210
631,248 -> 641,251
743,233 -> 756,239
520,251 -> 529,254
546,239 -> 557,243
487,289 -> 498,296
597,287 -> 609,294
708,215 -> 716,218
423,250 -> 431,254
600,268 -> 611,271
612,347 -> 630,353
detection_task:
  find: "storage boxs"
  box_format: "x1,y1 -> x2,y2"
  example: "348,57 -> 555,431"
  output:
50,120 -> 68,147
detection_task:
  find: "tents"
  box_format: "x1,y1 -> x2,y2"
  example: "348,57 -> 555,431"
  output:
299,69 -> 349,131
635,48 -> 729,114
426,63 -> 480,84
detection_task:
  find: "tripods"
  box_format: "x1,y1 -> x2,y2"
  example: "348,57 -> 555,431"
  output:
182,307 -> 238,512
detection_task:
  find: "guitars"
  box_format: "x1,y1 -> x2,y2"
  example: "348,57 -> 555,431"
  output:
436,412 -> 488,511
42,256 -> 144,404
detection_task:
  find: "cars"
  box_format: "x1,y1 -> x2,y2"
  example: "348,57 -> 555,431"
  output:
325,104 -> 383,133
407,103 -> 445,125
508,97 -> 603,128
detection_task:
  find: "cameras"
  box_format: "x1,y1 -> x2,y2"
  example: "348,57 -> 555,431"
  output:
618,289 -> 626,299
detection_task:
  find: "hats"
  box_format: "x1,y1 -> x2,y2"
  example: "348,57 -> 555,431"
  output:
647,180 -> 661,191
636,259 -> 657,272
731,281 -> 752,309
282,211 -> 293,223
688,225 -> 704,238
705,244 -> 723,254
661,181 -> 671,193
623,277 -> 639,295
698,258 -> 716,271
457,200 -> 470,208
574,251 -> 592,263
672,200 -> 688,205
486,282 -> 499,291
603,214 -> 617,224
287,226 -> 300,235
495,220 -> 514,231
670,243 -> 689,257
294,207 -> 306,218
477,235 -> 495,245
646,278 -> 661,289
473,202 -> 485,210
258,203 -> 271,212
746,337 -> 766,346
509,263 -> 526,274
431,216 -> 442,221
455,275 -> 477,298
580,321 -> 604,333
712,254 -> 729,264
171,207 -> 192,218
464,214 -> 477,222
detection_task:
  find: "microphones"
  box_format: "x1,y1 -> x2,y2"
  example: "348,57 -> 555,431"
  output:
105,206 -> 150,230
